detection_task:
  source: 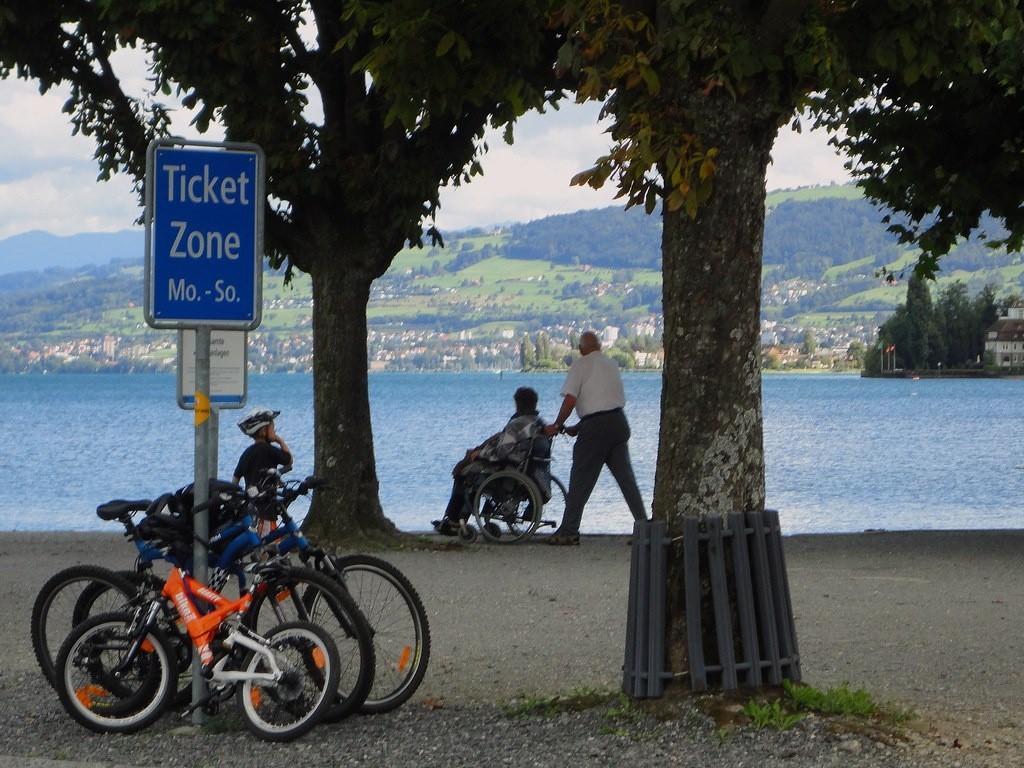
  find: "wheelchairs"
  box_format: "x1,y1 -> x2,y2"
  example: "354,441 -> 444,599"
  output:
434,418 -> 569,546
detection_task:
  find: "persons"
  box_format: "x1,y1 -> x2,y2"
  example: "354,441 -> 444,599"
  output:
232,408 -> 293,603
544,331 -> 648,546
431,386 -> 548,536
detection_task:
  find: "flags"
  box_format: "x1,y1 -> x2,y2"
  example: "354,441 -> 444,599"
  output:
891,346 -> 895,351
878,341 -> 882,350
886,346 -> 890,353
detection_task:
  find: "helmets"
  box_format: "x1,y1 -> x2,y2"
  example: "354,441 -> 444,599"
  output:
237,408 -> 281,435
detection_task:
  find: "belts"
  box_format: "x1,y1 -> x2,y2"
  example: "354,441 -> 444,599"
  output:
581,408 -> 620,422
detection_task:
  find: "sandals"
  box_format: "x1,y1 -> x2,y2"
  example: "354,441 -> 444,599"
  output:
432,517 -> 461,536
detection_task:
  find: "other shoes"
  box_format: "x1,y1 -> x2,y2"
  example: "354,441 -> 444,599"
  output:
543,524 -> 581,546
627,539 -> 633,546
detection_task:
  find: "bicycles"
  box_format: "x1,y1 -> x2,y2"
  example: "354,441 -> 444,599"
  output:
28,474 -> 431,744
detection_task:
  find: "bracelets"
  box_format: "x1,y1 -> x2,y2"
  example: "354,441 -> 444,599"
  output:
554,422 -> 560,430
